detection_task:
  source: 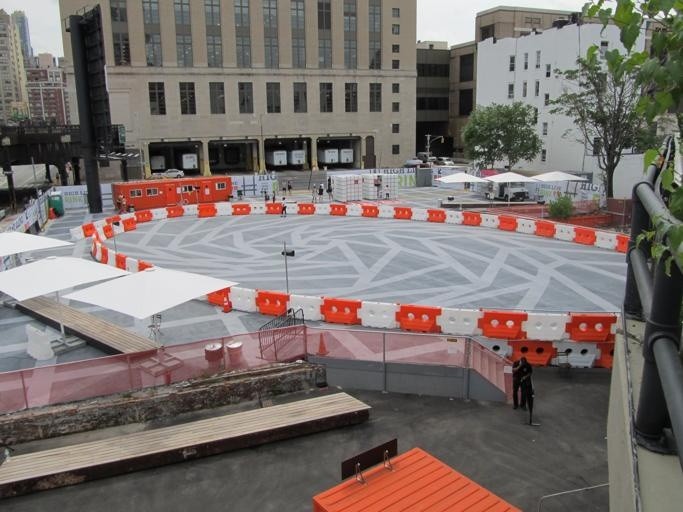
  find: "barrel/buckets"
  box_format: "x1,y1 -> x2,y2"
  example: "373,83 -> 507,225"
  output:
48,191 -> 64,215
204,342 -> 223,368
225,340 -> 244,366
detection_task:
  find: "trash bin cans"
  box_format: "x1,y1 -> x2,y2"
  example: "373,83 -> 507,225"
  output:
204,342 -> 223,369
226,340 -> 243,366
48,191 -> 64,216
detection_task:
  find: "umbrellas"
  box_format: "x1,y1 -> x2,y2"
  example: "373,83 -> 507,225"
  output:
482,171 -> 540,211
0,255 -> 132,343
529,170 -> 590,201
434,172 -> 490,210
0,231 -> 76,255
62,263 -> 240,345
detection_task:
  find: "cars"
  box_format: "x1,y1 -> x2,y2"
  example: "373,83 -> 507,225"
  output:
152,168 -> 183,179
406,152 -> 454,166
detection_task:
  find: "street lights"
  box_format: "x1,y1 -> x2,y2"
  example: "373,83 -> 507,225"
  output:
280,240 -> 294,294
255,111 -> 267,176
536,199 -> 545,219
110,218 -> 121,253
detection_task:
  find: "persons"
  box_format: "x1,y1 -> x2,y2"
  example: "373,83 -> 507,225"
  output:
318,186 -> 323,201
116,194 -> 123,209
325,184 -> 333,201
380,184 -> 391,200
287,180 -> 291,192
281,180 -> 286,195
511,356 -> 533,411
279,197 -> 287,218
121,195 -> 127,213
264,191 -> 269,204
272,191 -> 276,203
311,185 -> 317,204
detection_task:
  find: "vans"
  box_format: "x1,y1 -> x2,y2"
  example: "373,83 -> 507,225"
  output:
480,182 -> 528,202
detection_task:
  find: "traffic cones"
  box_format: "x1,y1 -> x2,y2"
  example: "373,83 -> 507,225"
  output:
315,334 -> 330,357
